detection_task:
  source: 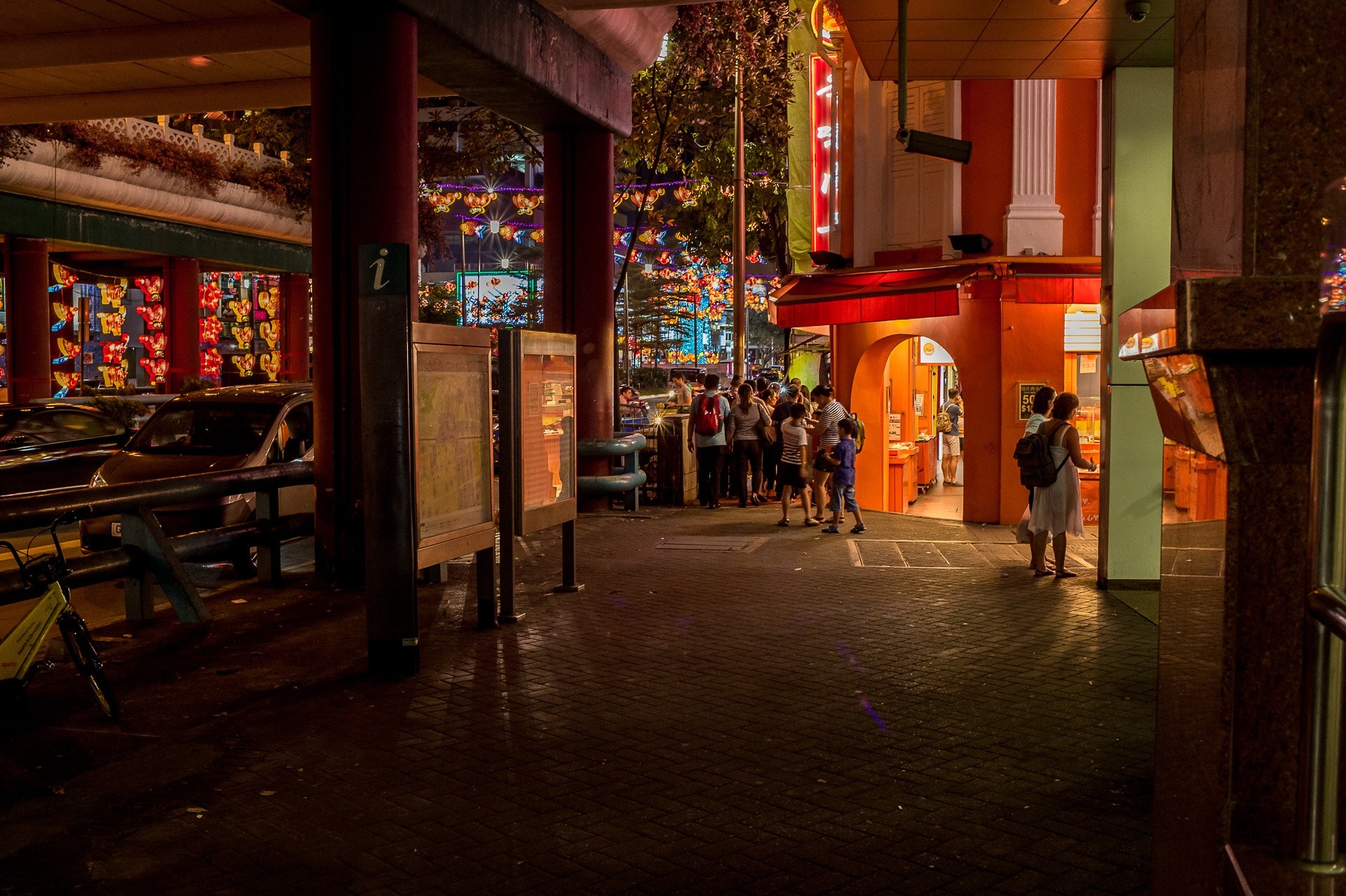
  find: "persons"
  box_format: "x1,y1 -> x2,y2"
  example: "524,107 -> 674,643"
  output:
665,371 -> 867,535
1022,385 -> 1098,579
937,388 -> 964,487
618,386 -> 634,417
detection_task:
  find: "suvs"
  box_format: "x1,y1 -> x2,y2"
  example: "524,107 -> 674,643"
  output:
80,382 -> 314,558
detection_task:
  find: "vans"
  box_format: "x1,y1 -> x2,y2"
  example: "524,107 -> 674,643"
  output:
667,367 -> 707,400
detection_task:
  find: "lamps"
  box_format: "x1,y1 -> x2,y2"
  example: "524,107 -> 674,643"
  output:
896,0 -> 973,165
808,250 -> 853,269
948,234 -> 993,254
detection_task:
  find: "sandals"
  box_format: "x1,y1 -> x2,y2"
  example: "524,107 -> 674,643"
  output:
805,517 -> 820,526
1034,567 -> 1056,576
778,518 -> 790,527
1055,567 -> 1078,578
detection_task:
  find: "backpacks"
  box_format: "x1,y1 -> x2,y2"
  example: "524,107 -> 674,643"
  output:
1013,421 -> 1070,488
839,403 -> 866,454
695,394 -> 724,437
936,404 -> 955,433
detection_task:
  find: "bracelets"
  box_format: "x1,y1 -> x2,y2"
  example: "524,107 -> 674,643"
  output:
675,403 -> 677,407
687,439 -> 693,442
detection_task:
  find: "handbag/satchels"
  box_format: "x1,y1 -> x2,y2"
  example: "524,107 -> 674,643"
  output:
1015,503 -> 1052,544
757,403 -> 776,446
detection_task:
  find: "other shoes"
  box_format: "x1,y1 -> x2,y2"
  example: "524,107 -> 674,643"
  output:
812,516 -> 825,524
850,522 -> 866,533
1030,559 -> 1056,570
821,525 -> 840,534
943,480 -> 950,485
1045,556 -> 1056,565
949,481 -> 963,487
697,476 -> 835,509
824,516 -> 845,523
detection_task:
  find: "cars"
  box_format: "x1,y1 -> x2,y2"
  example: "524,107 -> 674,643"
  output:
0,402 -> 142,522
756,365 -> 784,385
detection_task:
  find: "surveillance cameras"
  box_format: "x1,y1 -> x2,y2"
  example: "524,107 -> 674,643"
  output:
1125,1 -> 1151,23
